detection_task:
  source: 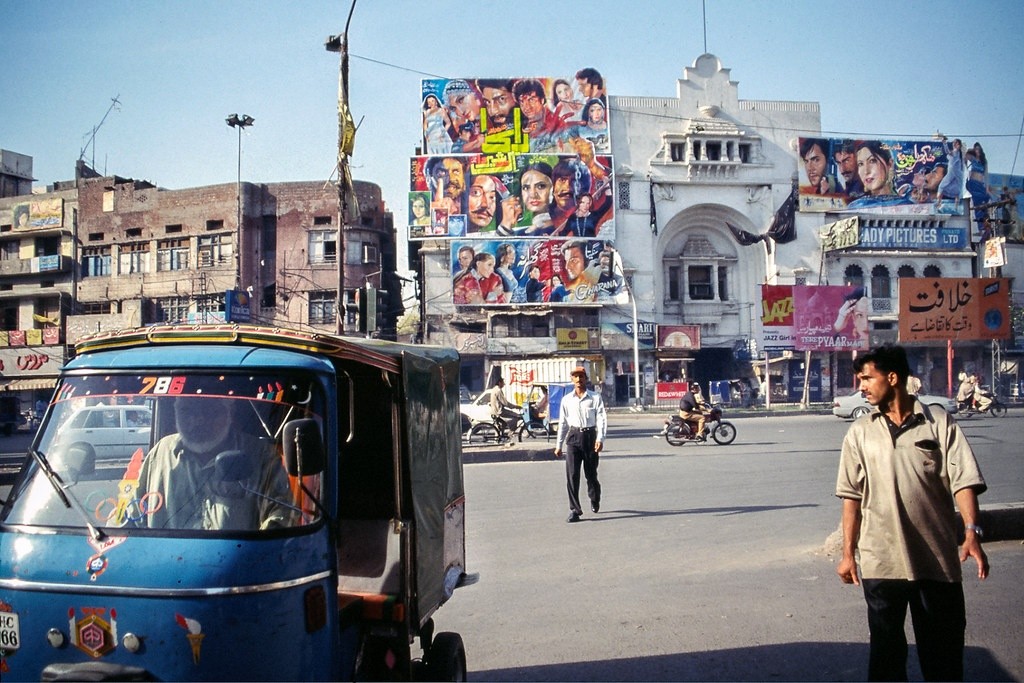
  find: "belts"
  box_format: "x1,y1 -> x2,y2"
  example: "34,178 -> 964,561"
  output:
570,425 -> 595,432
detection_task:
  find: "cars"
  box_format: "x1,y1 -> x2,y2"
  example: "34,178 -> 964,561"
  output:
831,389 -> 960,422
55,404 -> 152,474
461,389 -> 496,424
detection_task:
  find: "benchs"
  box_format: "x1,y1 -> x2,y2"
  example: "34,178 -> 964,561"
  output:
333,512 -> 405,623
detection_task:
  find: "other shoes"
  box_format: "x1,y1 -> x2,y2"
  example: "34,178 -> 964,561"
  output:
697,435 -> 706,442
591,502 -> 599,512
568,513 -> 579,522
508,431 -> 518,436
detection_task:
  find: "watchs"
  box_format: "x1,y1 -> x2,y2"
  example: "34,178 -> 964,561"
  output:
964,523 -> 984,538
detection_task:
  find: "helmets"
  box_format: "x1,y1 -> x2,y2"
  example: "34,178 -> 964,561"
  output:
174,395 -> 236,455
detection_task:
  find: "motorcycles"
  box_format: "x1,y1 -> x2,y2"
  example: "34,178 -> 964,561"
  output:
661,401 -> 735,445
958,390 -> 1007,418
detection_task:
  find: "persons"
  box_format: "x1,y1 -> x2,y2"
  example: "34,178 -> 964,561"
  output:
554,366 -> 608,521
531,386 -> 549,418
403,66 -> 630,314
826,287 -> 870,347
791,117 -> 1022,246
489,376 -> 524,444
905,360 -> 991,416
836,340 -> 990,683
730,382 -> 744,404
677,383 -> 709,442
126,383 -> 294,539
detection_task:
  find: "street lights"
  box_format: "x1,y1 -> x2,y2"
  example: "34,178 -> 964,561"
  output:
225,113 -> 254,291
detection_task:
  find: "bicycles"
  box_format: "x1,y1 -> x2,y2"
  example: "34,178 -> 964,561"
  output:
466,413 -> 551,444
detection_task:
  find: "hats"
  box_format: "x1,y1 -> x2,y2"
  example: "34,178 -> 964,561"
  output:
572,367 -> 586,375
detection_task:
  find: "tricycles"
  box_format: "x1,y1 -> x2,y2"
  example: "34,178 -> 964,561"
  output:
521,382 -> 596,435
0,324 -> 480,683
